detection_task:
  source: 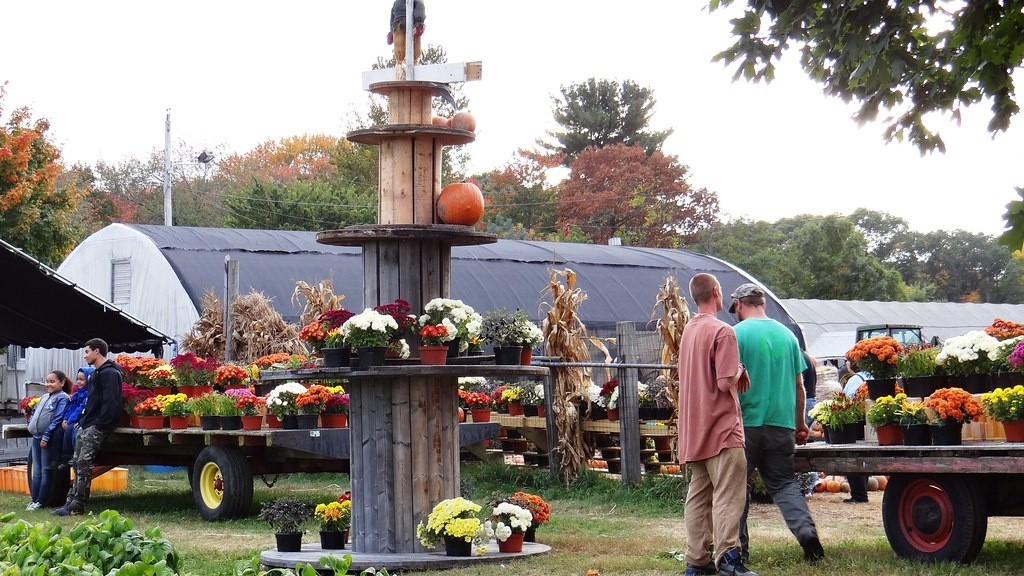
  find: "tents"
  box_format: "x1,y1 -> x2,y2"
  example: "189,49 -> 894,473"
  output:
27,224 -> 1024,402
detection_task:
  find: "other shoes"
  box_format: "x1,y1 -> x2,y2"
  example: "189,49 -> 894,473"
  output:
68,458 -> 74,466
801,534 -> 825,565
58,464 -> 69,470
51,507 -> 84,515
24,502 -> 44,511
843,497 -> 868,503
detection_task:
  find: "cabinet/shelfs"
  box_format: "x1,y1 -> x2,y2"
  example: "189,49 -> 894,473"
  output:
466,411 -> 686,466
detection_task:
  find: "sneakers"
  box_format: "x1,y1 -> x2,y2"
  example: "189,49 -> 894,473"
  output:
685,546 -> 719,576
718,546 -> 759,576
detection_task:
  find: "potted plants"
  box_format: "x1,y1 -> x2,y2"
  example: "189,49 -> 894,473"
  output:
753,474 -> 772,503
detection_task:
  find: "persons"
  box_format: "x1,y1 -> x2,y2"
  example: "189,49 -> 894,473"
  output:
26,338 -> 121,515
676,273 -> 876,576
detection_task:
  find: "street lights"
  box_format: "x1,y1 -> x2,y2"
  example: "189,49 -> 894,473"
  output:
163,149 -> 216,227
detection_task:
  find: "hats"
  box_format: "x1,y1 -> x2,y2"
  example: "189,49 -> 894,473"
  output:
728,283 -> 764,314
837,365 -> 850,382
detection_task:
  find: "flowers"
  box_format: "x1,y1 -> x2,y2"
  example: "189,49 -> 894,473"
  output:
458,378 -> 673,409
20,395 -> 41,421
114,350 -> 349,417
808,317 -> 1024,433
258,491 -> 351,533
416,491 -> 551,556
297,298 -> 544,349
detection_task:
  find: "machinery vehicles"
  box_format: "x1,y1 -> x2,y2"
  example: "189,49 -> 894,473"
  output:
839,323 -> 924,389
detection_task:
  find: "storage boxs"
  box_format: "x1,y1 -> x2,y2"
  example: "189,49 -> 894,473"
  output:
0,465 -> 129,495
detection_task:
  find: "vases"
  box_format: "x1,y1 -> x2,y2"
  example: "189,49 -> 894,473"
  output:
495,401 -> 675,419
822,371 -> 1024,446
498,531 -> 524,552
443,536 -> 472,556
525,526 -> 535,543
119,384 -> 349,429
274,524 -> 351,552
595,436 -> 672,474
321,337 -> 533,369
463,409 -> 468,423
501,430 -> 527,452
470,407 -> 491,421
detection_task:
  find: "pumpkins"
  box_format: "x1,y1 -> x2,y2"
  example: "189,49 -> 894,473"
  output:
587,459 -> 608,468
437,180 -> 484,226
459,405 -> 464,424
810,421 -> 887,493
660,465 -> 680,473
432,111 -> 477,131
